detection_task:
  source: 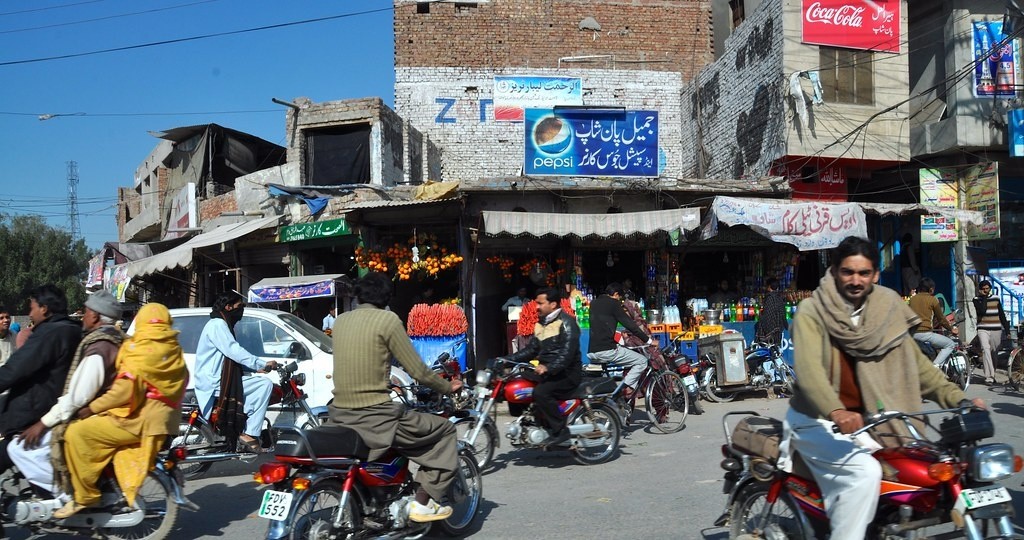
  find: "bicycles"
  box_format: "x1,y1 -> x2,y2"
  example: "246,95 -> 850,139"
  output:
581,343 -> 689,434
913,309 -> 970,392
1007,339 -> 1024,393
964,345 -> 1012,384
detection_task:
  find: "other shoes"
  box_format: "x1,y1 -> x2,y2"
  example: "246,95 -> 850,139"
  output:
54,501 -> 100,518
985,378 -> 995,386
540,427 -> 571,448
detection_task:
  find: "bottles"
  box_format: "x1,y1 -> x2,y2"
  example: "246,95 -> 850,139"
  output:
712,289 -> 812,322
575,293 -> 647,328
686,299 -> 709,317
664,305 -> 681,325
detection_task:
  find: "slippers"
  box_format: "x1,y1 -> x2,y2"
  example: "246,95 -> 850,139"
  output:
239,434 -> 262,453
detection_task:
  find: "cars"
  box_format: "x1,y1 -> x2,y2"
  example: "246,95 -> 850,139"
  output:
124,306 -> 417,444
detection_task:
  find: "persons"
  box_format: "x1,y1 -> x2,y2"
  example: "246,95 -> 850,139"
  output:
193,291 -> 279,455
494,288 -> 583,451
6,289 -> 125,507
53,302 -> 189,519
972,281 -> 1011,386
322,301 -> 338,331
784,235 -> 988,540
754,277 -> 788,377
619,279 -> 635,300
502,284 -> 531,311
0,283 -> 80,504
894,275 -> 960,371
586,282 -> 659,400
707,278 -> 743,310
327,273 -> 463,522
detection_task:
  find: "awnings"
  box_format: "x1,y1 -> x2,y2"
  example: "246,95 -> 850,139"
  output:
478,205 -> 708,240
107,215 -> 289,280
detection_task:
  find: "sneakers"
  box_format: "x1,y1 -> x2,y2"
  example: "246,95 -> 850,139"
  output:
409,499 -> 454,522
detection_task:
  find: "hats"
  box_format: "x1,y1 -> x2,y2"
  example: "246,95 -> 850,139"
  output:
85,289 -> 123,318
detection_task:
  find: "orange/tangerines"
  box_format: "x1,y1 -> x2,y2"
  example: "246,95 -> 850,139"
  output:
355,236 -> 464,280
486,255 -> 567,287
439,298 -> 458,306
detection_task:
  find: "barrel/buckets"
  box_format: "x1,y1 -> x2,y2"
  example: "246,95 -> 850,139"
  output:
647,310 -> 663,325
703,309 -> 722,325
408,332 -> 466,373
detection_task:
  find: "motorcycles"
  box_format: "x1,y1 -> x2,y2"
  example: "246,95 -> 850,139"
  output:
944,331 -> 967,389
699,399 -> 1024,540
253,382 -> 483,540
699,332 -> 796,403
429,338 -> 472,397
661,333 -> 703,415
156,361 -> 330,480
445,356 -> 626,475
0,444 -> 200,540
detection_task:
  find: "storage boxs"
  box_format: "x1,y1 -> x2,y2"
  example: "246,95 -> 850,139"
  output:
647,323 -> 698,375
